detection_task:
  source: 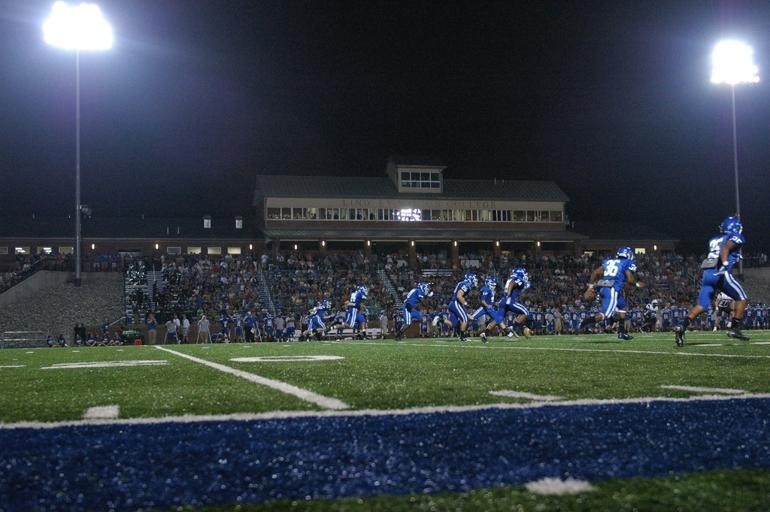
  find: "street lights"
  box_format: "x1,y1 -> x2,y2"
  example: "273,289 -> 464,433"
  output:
707,37 -> 762,280
40,2 -> 115,286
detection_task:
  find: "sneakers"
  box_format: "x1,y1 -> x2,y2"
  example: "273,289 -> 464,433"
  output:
458,325 -> 521,343
726,327 -> 749,341
577,318 -> 585,329
432,315 -> 439,326
617,333 -> 633,341
675,324 -> 684,348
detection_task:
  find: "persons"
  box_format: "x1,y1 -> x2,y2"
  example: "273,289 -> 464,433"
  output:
1,244 -> 770,345
675,214 -> 749,346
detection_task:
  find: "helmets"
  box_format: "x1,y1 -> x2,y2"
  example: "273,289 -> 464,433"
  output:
464,272 -> 478,289
323,299 -> 332,309
417,282 -> 431,296
717,215 -> 745,234
356,284 -> 368,296
510,268 -> 528,279
615,246 -> 633,260
485,277 -> 496,291
